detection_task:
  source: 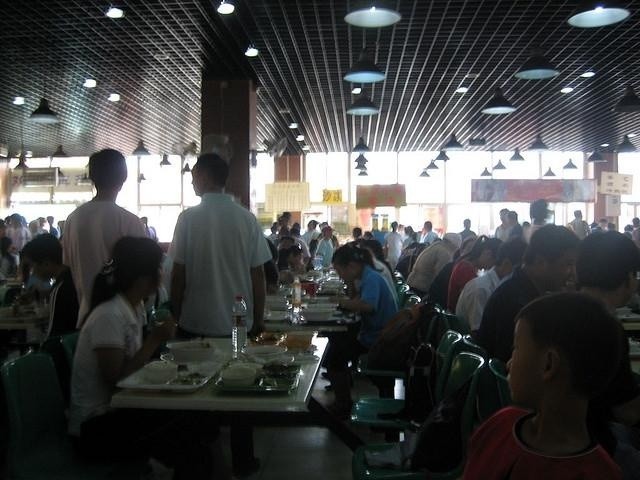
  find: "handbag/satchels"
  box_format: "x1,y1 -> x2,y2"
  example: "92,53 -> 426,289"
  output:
411,396 -> 463,472
370,302 -> 433,370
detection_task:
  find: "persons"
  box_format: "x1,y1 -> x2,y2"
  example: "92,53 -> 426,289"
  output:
22,232 -> 80,348
59,148 -> 147,303
267,211 -> 339,296
461,292 -> 638,480
68,235 -> 163,440
0,212 -> 59,283
160,152 -> 272,342
141,216 -> 156,241
324,198 -> 639,362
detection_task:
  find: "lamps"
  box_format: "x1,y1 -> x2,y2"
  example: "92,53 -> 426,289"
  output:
420,170 -> 430,177
510,147 -> 525,160
160,154 -> 171,165
346,89 -> 379,116
614,86 -> 640,112
352,137 -> 370,152
435,151 -> 449,160
52,145 -> 71,157
354,162 -> 367,169
182,163 -> 191,171
357,170 -> 368,176
441,134 -> 464,148
514,54 -> 558,80
343,50 -> 386,84
427,160 -> 439,169
544,167 -> 556,176
587,149 -> 608,162
27,98 -> 58,119
132,141 -> 151,155
343,1 -> 402,28
563,158 -> 577,169
527,135 -> 548,149
567,0 -> 630,28
480,167 -> 492,176
354,153 -> 368,162
480,88 -> 517,115
616,135 -> 637,152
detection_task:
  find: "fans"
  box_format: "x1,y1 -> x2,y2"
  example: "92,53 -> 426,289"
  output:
172,142 -> 201,160
252,138 -> 288,167
201,133 -> 234,166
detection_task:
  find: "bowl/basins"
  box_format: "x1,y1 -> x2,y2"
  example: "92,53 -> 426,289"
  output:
144,365 -> 179,383
239,346 -> 287,360
220,366 -> 257,386
166,342 -> 216,360
302,309 -> 332,321
264,310 -> 289,332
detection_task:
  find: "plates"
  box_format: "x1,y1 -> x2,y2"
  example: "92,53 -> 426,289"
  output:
305,318 -> 339,324
159,348 -> 223,362
257,354 -> 295,367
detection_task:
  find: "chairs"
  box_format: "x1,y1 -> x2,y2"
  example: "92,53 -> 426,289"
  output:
356,295 -> 423,349
358,304 -> 441,380
492,160 -> 507,169
462,335 -> 488,359
396,283 -> 410,304
1,354 -> 76,480
442,309 -> 468,337
350,330 -> 462,430
489,358 -> 528,408
352,352 -> 486,477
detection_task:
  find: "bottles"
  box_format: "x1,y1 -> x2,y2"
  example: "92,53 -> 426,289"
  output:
230,294 -> 247,353
